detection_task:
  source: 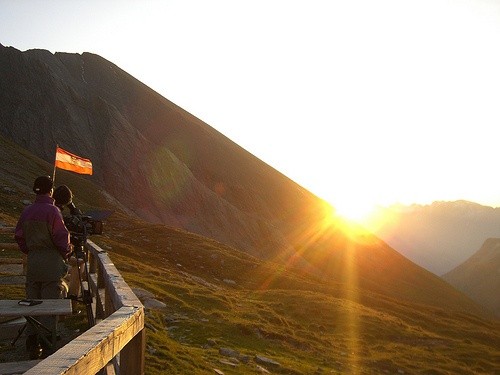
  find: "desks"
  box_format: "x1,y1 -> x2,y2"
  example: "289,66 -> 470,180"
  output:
0,298 -> 73,353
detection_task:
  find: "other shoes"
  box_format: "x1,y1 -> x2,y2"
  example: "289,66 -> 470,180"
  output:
30,348 -> 42,360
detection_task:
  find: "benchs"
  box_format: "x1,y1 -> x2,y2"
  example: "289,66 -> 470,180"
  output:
0,360 -> 43,375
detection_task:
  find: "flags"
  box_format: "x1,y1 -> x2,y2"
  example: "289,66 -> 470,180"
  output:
55,147 -> 93,175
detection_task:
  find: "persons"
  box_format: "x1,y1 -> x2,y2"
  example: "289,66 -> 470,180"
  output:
51,185 -> 86,334
15,176 -> 72,358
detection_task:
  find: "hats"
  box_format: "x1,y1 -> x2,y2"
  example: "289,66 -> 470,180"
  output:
33,176 -> 55,193
53,185 -> 71,202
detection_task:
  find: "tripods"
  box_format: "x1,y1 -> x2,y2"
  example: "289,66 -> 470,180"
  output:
13,240 -> 91,346
